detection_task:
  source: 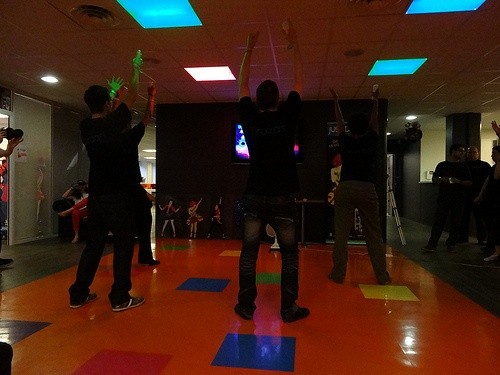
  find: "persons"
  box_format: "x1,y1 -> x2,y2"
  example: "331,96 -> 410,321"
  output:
328,85 -> 393,285
59,50 -> 160,312
0,127 -> 24,269
420,121 -> 500,262
234,21 -> 310,322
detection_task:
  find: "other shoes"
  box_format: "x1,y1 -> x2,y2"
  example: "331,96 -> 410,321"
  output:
480,246 -> 496,253
448,247 -> 458,253
137,259 -> 160,266
58,212 -> 67,217
421,247 -> 437,254
72,236 -> 79,244
0,257 -> 14,268
282,306 -> 310,322
477,240 -> 484,246
379,276 -> 391,285
328,273 -> 343,284
234,303 -> 254,321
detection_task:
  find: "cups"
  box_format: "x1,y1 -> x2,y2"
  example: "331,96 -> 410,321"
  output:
448,178 -> 453,183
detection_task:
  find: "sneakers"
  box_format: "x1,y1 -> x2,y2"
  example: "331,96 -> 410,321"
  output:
69,293 -> 98,308
111,296 -> 145,312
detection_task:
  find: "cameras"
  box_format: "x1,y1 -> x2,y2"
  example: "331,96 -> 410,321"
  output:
3,128 -> 24,140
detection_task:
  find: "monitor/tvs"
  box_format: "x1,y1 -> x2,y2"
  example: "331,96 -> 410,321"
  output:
230,119 -> 305,164
